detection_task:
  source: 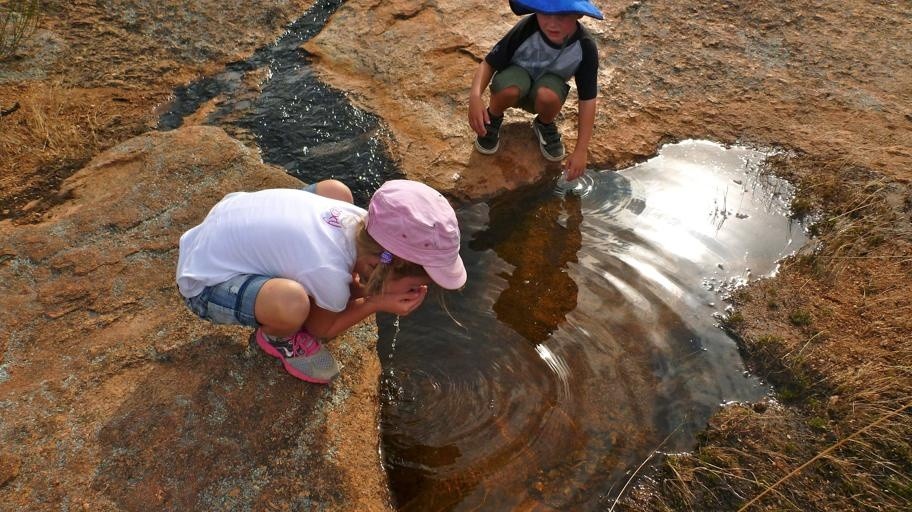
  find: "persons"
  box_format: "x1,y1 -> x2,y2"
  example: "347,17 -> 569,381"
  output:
174,178 -> 469,386
467,0 -> 605,183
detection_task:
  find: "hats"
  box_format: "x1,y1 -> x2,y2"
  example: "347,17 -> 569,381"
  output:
364,179 -> 468,290
509,0 -> 604,20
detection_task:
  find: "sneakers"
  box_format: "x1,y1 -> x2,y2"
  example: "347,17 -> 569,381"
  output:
475,107 -> 504,154
256,327 -> 340,384
532,115 -> 565,162
